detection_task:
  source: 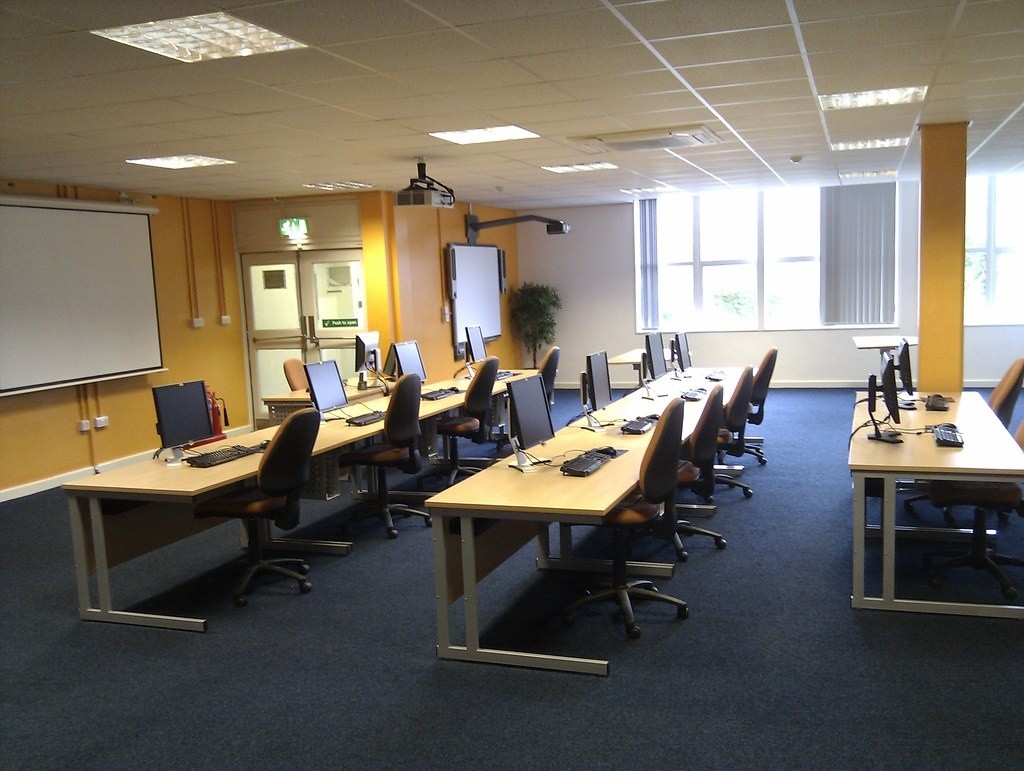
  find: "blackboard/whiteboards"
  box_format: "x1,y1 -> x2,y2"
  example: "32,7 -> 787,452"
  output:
447,241 -> 502,347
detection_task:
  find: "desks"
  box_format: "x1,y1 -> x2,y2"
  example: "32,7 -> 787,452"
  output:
425,366 -> 759,675
852,335 -> 918,391
60,369 -> 539,632
849,391 -> 1024,621
261,377 -> 398,428
607,348 -> 678,398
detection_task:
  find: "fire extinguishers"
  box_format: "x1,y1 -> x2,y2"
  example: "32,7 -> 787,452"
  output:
211,392 -> 229,436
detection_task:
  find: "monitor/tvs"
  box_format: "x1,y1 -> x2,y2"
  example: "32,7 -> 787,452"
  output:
641,332 -> 669,400
465,326 -> 487,377
304,359 -> 349,424
670,332 -> 692,380
506,372 -> 555,473
891,338 -> 918,410
394,340 -> 428,382
152,379 -> 215,467
382,342 -> 396,382
580,350 -> 612,434
867,352 -> 903,444
355,330 -> 384,387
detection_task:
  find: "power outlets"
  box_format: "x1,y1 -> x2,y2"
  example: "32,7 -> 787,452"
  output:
79,420 -> 90,432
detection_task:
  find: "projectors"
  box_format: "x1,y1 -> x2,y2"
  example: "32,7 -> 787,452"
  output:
546,224 -> 570,234
392,191 -> 454,209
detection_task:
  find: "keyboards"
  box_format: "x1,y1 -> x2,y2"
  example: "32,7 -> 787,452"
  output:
186,444 -> 256,468
494,371 -> 513,381
925,397 -> 949,411
681,388 -> 706,401
933,427 -> 964,447
706,372 -> 725,381
621,417 -> 657,434
346,410 -> 386,426
560,450 -> 611,477
420,388 -> 456,400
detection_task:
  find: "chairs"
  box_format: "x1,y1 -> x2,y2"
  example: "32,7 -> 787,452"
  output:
193,407 -> 321,608
338,373 -> 433,539
904,357 -> 1024,523
496,346 -> 560,455
624,345 -> 780,561
562,398 -> 691,639
284,358 -> 309,392
921,415 -> 1024,601
416,355 -> 499,493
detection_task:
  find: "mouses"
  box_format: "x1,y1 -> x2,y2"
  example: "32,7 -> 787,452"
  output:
447,387 -> 459,392
646,414 -> 660,420
929,394 -> 943,398
698,388 -> 707,392
596,446 -> 617,455
259,440 -> 271,448
935,423 -> 956,428
